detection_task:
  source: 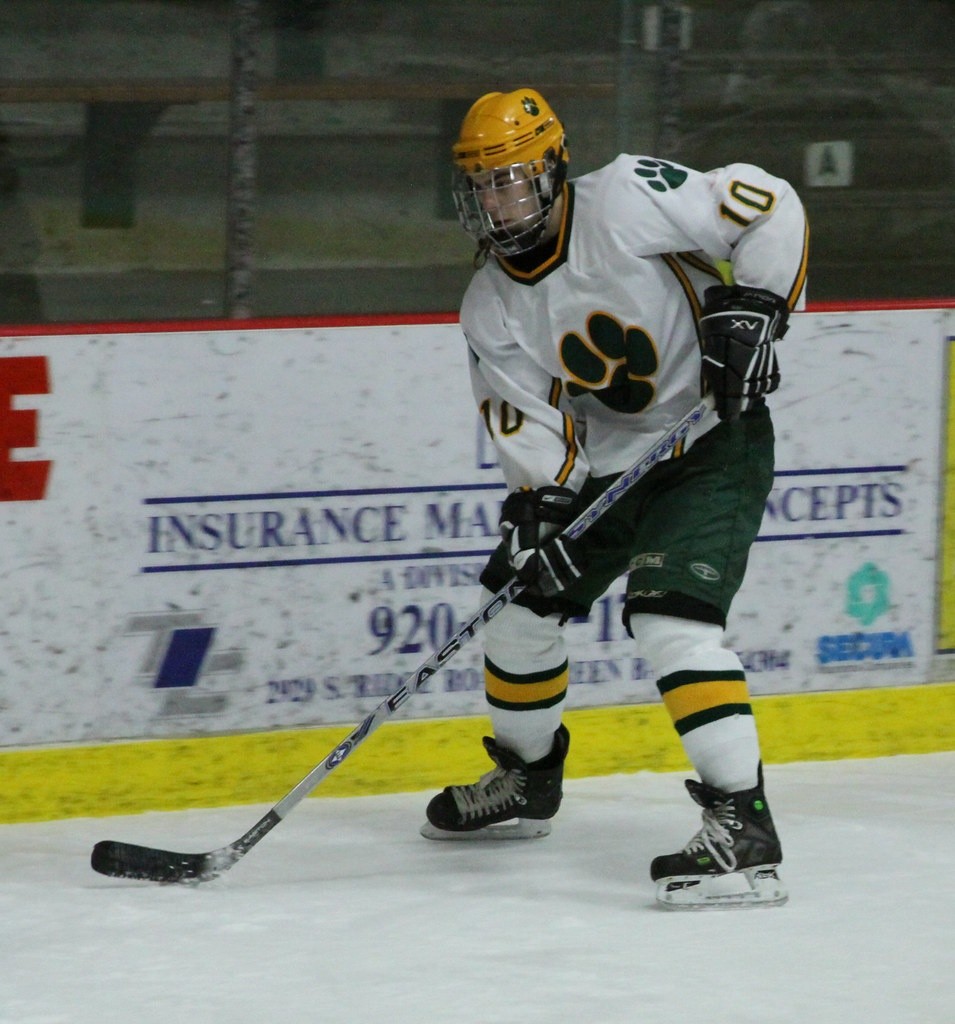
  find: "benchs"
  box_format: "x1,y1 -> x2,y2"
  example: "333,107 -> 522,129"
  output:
0,36 -> 954,232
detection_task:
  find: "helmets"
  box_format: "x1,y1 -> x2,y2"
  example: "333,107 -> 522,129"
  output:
451,88 -> 570,257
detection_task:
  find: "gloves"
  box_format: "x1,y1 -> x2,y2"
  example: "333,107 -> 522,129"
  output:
498,486 -> 586,598
699,284 -> 790,423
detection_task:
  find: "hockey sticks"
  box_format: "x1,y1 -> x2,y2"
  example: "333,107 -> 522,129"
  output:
89,393 -> 715,889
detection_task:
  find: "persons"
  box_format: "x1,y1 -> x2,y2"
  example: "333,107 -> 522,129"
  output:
419,89 -> 811,909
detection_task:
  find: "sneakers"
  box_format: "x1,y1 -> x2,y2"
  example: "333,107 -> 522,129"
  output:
650,760 -> 789,910
419,723 -> 570,840
